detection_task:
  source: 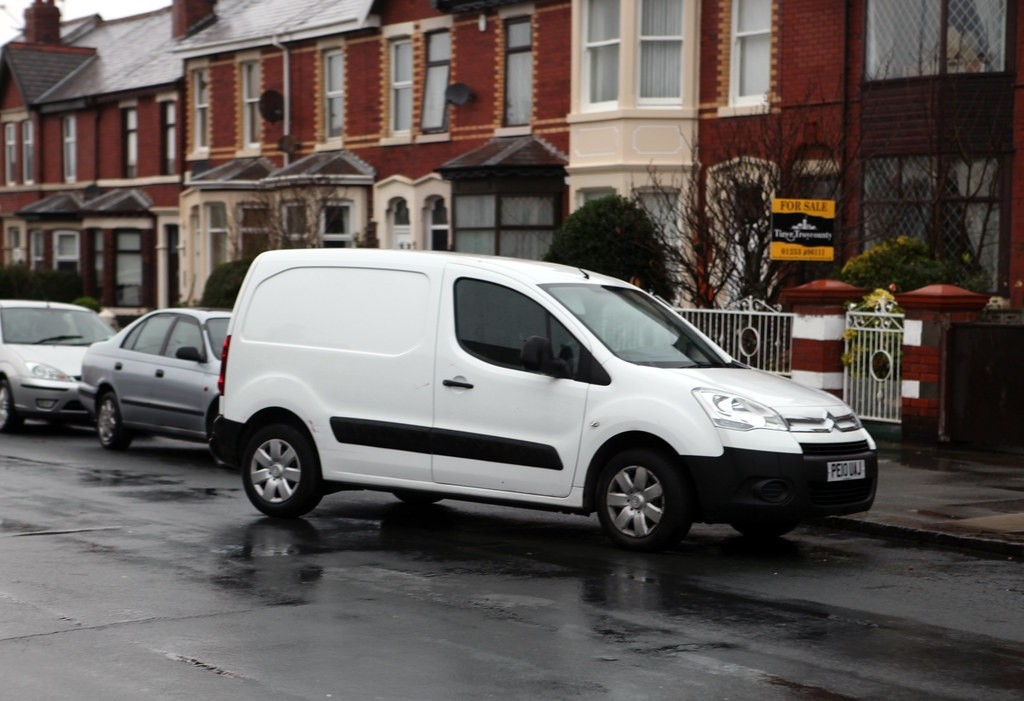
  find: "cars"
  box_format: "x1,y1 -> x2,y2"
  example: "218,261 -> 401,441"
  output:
0,300 -> 118,435
77,308 -> 233,452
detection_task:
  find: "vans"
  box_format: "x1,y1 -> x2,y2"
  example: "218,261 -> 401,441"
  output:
208,247 -> 879,555
